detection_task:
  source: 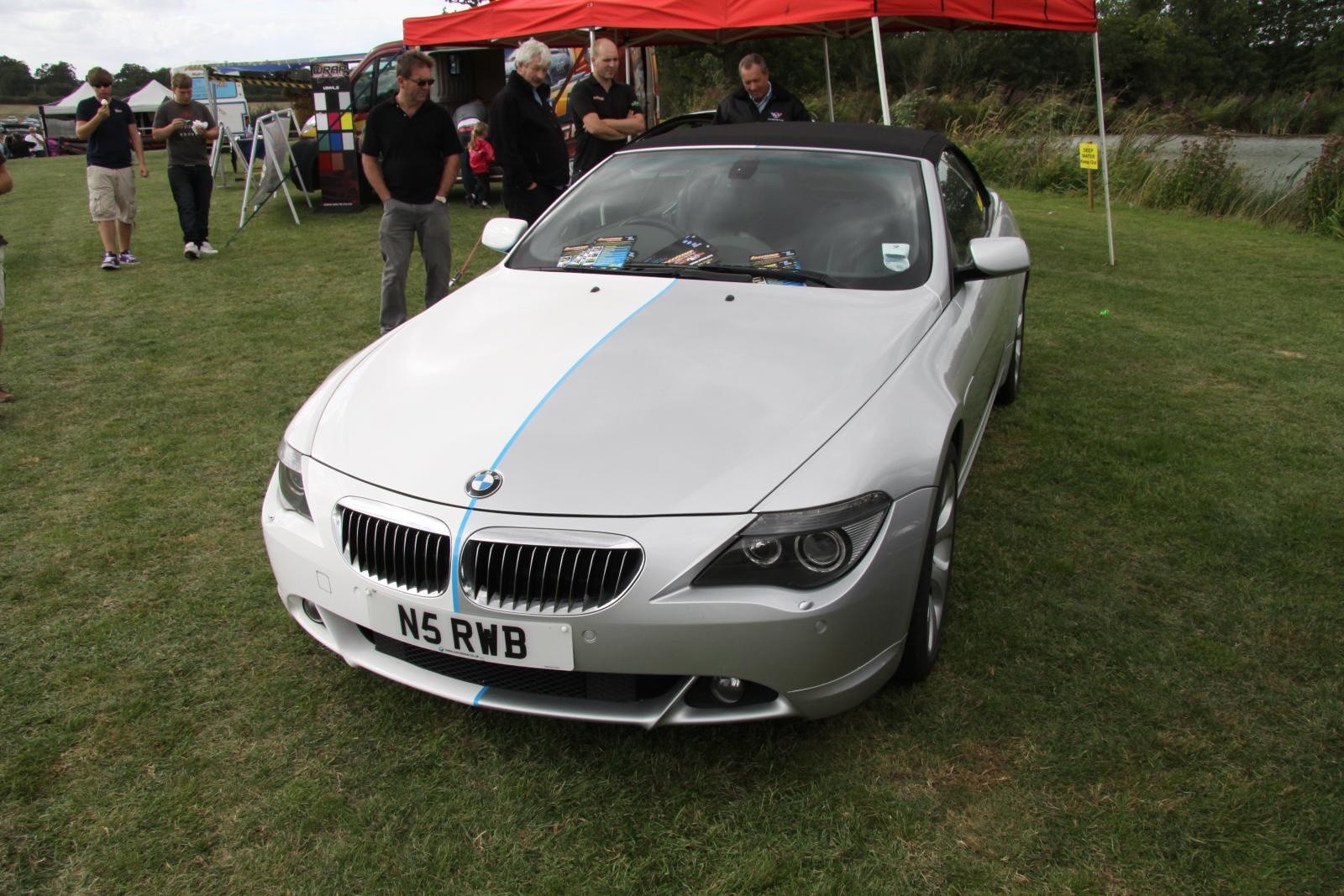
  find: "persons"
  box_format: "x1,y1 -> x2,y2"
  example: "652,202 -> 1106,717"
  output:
569,39 -> 646,185
0,149 -> 16,402
452,97 -> 487,200
359,49 -> 466,337
712,53 -> 811,125
0,126 -> 47,159
151,73 -> 219,260
487,38 -> 572,253
466,121 -> 495,210
75,67 -> 149,270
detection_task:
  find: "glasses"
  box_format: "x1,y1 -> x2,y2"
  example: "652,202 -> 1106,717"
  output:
94,84 -> 109,89
409,78 -> 435,86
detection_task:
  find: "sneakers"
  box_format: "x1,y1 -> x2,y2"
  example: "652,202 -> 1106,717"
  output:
118,250 -> 141,264
184,242 -> 200,260
101,252 -> 120,268
200,242 -> 218,253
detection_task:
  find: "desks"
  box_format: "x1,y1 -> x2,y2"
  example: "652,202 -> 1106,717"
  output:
231,138 -> 266,182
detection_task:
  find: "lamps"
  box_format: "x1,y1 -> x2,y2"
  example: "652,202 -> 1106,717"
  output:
447,54 -> 463,77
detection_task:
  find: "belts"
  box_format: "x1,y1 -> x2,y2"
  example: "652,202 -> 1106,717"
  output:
457,127 -> 473,134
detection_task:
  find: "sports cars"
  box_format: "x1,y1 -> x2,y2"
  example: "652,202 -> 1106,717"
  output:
259,114 -> 1037,735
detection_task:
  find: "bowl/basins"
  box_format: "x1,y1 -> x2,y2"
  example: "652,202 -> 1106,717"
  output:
238,134 -> 245,138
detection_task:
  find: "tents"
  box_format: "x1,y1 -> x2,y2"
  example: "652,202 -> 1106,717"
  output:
401,0 -> 1117,268
38,80 -> 100,157
120,77 -> 176,150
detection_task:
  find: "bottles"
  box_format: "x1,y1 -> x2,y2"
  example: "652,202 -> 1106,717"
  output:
247,124 -> 252,138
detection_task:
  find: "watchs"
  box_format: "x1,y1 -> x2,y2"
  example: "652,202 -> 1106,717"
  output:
434,195 -> 447,204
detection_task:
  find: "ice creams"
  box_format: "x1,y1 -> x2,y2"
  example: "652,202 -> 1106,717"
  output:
100,99 -> 109,107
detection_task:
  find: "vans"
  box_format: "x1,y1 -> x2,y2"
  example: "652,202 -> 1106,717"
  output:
168,61 -> 254,148
287,39 -> 664,200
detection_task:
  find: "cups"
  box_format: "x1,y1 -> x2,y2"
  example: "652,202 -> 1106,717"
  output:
246,134 -> 250,138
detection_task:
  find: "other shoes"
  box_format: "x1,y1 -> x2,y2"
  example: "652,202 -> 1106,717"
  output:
466,195 -> 475,207
482,203 -> 489,209
